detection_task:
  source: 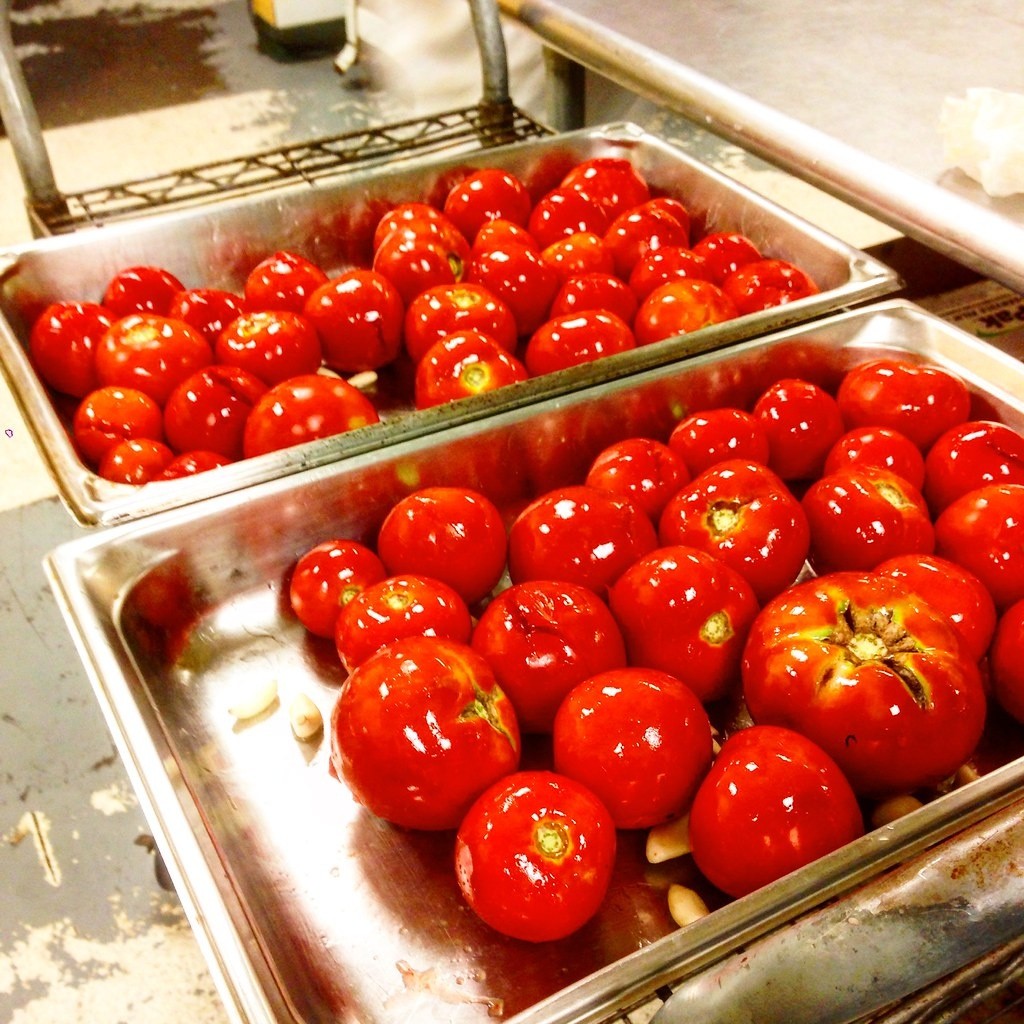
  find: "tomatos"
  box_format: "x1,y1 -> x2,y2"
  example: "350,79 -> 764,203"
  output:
29,159 -> 1024,945
